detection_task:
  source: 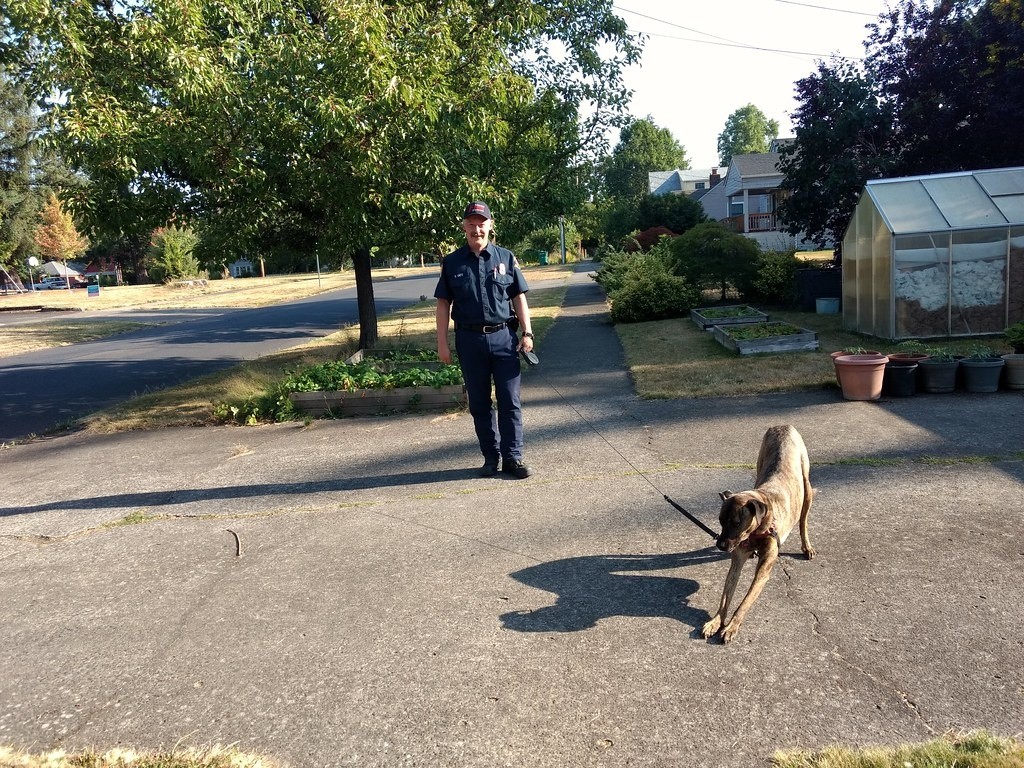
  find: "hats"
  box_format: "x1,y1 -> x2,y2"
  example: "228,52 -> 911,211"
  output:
464,201 -> 491,220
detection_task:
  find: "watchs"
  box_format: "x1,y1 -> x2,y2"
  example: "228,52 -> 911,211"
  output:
522,332 -> 535,341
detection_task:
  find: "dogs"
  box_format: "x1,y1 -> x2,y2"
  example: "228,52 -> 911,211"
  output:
701,425 -> 818,643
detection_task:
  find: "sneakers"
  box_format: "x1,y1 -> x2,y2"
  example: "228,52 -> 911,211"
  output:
479,460 -> 498,477
501,460 -> 533,478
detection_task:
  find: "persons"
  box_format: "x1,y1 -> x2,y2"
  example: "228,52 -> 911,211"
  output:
434,201 -> 537,480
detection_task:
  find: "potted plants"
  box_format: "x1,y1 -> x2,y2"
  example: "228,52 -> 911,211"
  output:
830,323 -> 1024,401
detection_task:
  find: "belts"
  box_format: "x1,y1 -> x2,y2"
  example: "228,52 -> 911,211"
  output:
460,323 -> 510,333
